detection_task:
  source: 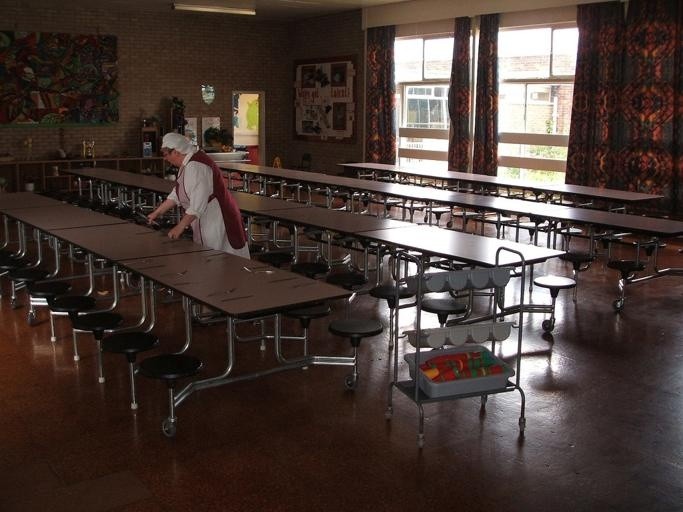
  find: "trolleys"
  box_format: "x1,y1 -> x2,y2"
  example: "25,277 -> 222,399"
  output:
381,246 -> 529,447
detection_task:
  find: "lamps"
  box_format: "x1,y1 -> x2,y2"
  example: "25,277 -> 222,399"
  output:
170,0 -> 260,18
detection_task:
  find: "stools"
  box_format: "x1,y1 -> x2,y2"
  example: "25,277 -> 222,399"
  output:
2,163 -> 681,450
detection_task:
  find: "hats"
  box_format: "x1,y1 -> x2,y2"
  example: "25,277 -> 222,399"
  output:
161,133 -> 192,154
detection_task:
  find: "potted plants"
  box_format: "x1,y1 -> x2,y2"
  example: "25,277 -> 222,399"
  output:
203,126 -> 234,152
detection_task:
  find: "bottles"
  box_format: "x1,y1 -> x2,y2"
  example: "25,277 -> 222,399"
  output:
420,350 -> 507,384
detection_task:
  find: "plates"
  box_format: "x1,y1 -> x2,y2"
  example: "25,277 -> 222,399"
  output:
213,159 -> 251,163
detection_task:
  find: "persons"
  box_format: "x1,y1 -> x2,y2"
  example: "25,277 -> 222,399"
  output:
145,132 -> 251,329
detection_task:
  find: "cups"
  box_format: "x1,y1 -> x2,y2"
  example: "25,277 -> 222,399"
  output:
51,165 -> 58,177
24,183 -> 33,192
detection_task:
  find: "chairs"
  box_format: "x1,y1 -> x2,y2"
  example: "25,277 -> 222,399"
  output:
288,154 -> 312,171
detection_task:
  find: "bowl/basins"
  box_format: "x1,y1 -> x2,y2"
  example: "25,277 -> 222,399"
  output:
205,151 -> 248,160
204,147 -> 218,153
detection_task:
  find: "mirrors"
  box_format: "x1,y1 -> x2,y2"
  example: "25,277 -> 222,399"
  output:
199,84 -> 217,106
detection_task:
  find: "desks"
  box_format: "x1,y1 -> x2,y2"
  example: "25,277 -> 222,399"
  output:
200,149 -> 249,164
